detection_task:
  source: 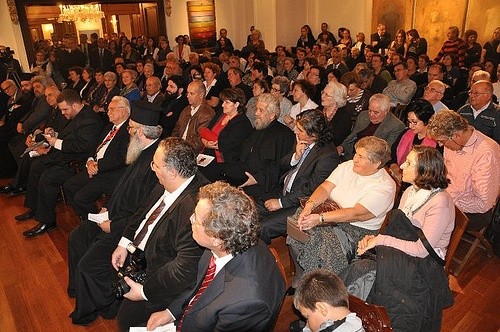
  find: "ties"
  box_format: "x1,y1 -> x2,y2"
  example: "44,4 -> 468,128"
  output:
133,200 -> 166,246
95,128 -> 120,155
100,50 -> 103,59
176,257 -> 216,332
84,44 -> 87,55
282,148 -> 310,196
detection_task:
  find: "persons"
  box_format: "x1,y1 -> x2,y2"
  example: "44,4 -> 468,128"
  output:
426,109 -> 500,232
0,22 -> 500,332
292,267 -> 367,332
338,145 -> 457,332
146,180 -> 286,332
110,136 -> 213,332
14,89 -> 103,238
67,102 -> 163,326
287,136 -> 397,298
61,96 -> 135,221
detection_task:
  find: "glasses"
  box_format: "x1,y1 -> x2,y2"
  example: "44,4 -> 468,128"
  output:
468,90 -> 488,97
407,120 -> 419,125
424,86 -> 442,95
270,86 -> 284,92
434,131 -> 456,146
321,90 -> 332,98
3,84 -> 12,92
150,161 -> 171,171
393,69 -> 405,74
106,106 -> 123,113
403,160 -> 418,167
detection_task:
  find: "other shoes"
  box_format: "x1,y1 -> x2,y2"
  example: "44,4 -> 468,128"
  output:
288,319 -> 305,332
286,286 -> 295,296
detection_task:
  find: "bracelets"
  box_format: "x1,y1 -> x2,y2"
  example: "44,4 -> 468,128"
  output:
305,200 -> 317,208
318,212 -> 325,226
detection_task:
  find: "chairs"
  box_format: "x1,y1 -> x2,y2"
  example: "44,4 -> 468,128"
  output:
271,198 -> 498,332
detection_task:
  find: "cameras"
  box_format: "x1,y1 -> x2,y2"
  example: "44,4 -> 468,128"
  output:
112,243 -> 148,298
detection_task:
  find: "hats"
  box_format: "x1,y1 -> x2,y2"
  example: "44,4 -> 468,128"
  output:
21,73 -> 35,81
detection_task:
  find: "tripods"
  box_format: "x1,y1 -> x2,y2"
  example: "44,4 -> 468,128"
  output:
6,68 -> 21,88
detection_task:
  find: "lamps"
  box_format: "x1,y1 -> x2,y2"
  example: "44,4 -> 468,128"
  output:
58,3 -> 104,23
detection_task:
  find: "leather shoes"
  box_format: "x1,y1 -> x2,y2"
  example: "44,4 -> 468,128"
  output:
15,210 -> 35,220
23,220 -> 56,237
0,184 -> 26,195
